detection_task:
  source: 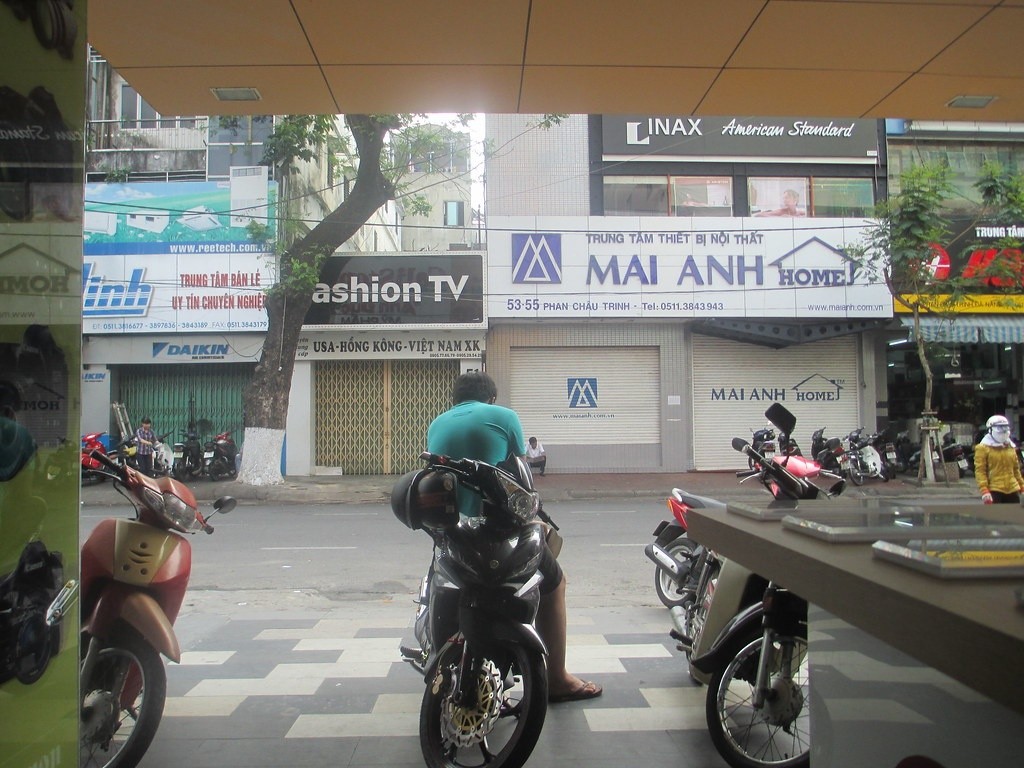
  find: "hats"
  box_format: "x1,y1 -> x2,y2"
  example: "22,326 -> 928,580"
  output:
141,417 -> 151,423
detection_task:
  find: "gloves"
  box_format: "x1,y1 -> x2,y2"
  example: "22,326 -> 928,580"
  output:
1019,487 -> 1024,494
982,494 -> 993,504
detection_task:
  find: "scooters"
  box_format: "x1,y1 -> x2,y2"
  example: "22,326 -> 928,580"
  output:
80,427 -> 239,482
644,402 -> 1024,768
78,450 -> 239,768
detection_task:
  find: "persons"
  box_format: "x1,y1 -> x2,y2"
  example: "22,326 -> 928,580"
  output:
973,415 -> 1024,504
427,372 -> 603,702
526,436 -> 547,477
753,189 -> 805,217
135,417 -> 160,476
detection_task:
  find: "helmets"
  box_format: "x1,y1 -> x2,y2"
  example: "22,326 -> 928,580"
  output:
391,468 -> 459,530
986,415 -> 1009,427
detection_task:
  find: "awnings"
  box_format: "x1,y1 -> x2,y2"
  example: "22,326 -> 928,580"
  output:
899,314 -> 1024,344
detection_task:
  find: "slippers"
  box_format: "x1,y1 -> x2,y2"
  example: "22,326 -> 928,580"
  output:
547,679 -> 602,704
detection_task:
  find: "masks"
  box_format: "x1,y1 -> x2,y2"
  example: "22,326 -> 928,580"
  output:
991,431 -> 1010,442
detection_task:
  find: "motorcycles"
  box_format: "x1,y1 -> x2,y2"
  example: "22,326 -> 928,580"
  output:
404,446 -> 568,768
